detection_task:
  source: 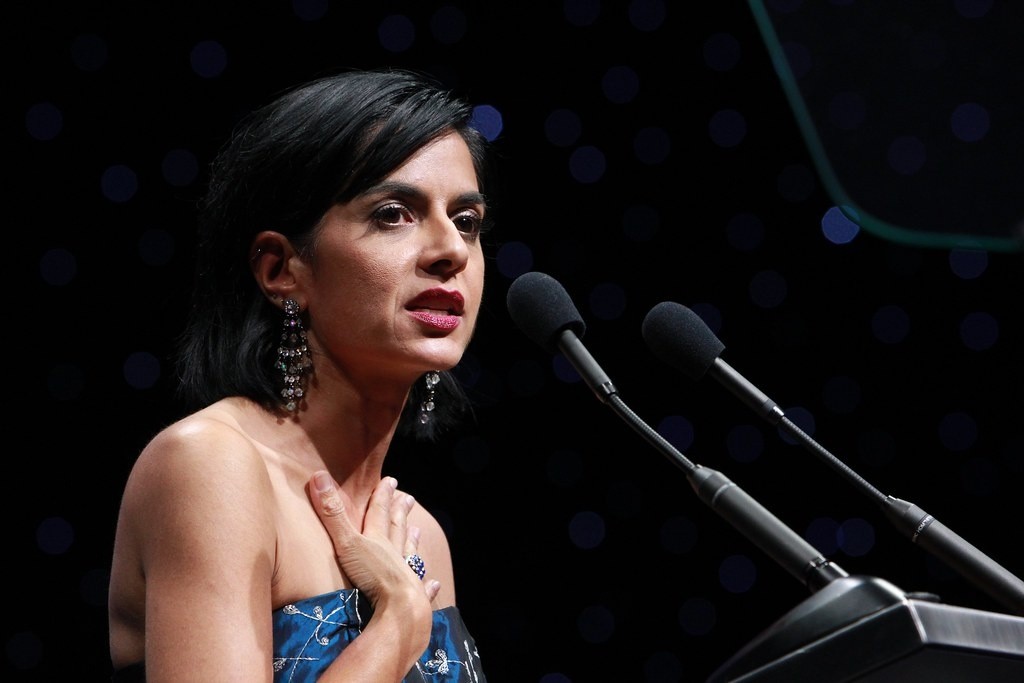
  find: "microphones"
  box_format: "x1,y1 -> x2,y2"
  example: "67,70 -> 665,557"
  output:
642,303 -> 1024,617
507,272 -> 939,683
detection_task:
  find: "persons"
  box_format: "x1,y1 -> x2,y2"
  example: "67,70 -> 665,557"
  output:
110,75 -> 486,683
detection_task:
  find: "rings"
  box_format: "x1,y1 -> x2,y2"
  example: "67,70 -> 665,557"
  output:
406,555 -> 425,580
